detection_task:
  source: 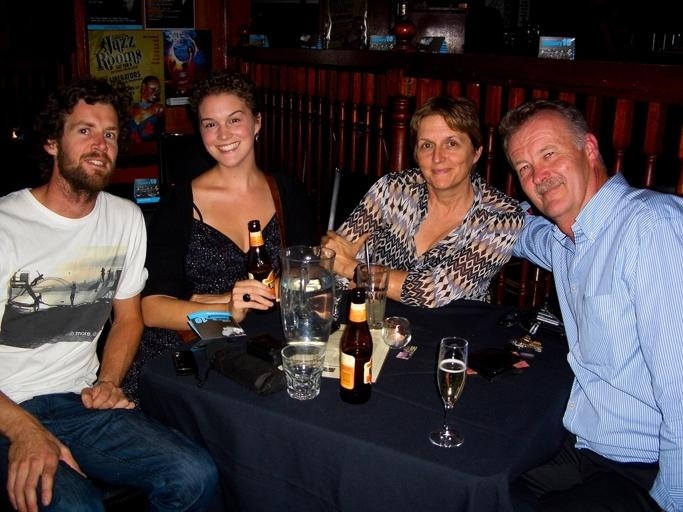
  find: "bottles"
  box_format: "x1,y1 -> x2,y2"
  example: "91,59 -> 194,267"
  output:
244,219 -> 277,316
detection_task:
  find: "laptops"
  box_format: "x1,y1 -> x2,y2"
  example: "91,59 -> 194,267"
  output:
418,36 -> 445,53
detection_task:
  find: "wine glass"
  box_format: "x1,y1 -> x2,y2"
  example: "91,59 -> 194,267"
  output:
429,335 -> 470,450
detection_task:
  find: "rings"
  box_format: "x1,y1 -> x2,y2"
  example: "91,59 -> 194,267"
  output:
242,294 -> 252,302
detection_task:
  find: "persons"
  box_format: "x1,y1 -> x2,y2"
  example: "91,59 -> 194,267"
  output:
0,72 -> 219,509
121,76 -> 171,159
316,94 -> 521,308
494,99 -> 682,509
139,73 -> 317,337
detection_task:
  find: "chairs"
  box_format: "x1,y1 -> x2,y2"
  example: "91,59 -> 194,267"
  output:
328,168 -> 381,232
93,480 -> 134,512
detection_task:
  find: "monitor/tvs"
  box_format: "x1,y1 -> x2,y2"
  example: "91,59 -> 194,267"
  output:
369,35 -> 396,51
537,36 -> 576,60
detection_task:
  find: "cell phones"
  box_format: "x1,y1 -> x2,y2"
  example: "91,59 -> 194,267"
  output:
171,350 -> 199,376
133,178 -> 162,212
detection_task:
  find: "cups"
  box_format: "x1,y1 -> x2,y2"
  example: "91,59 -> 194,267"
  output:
279,243 -> 413,402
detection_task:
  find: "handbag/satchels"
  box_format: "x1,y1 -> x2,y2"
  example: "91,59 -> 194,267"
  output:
211,333 -> 287,396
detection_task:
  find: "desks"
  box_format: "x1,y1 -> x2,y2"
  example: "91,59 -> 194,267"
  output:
136,299 -> 573,511
